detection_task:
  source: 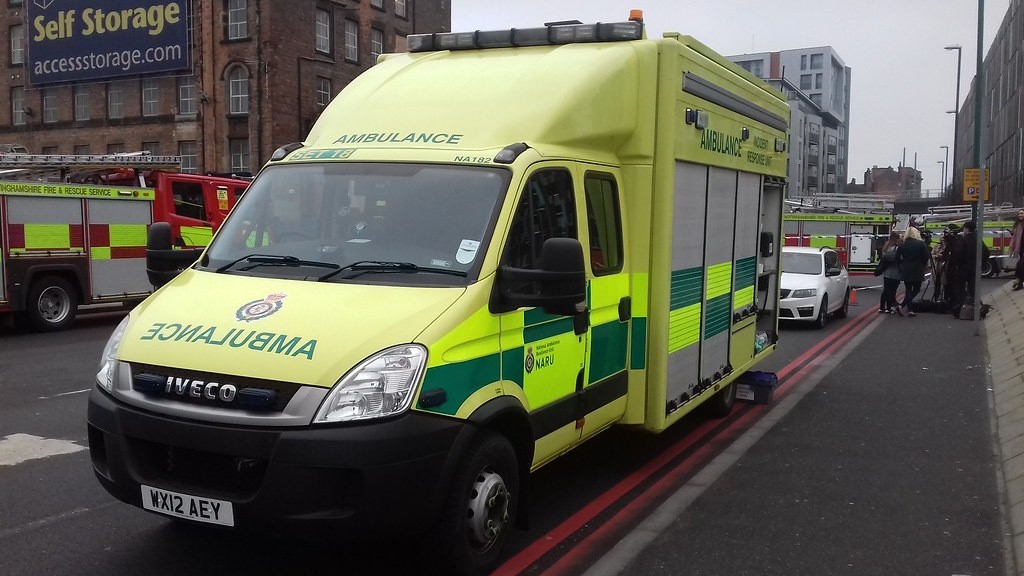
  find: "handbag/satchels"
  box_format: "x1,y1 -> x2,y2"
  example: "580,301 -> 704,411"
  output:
958,301 -> 994,321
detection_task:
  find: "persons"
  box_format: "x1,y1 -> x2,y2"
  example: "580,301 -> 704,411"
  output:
1008,209 -> 1024,291
879,221 -> 989,319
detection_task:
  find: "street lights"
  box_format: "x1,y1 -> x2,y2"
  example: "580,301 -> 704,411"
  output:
936,161 -> 944,205
946,110 -> 960,203
940,145 -> 948,206
944,43 -> 962,205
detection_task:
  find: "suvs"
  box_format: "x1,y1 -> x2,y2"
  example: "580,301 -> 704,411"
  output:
778,244 -> 851,329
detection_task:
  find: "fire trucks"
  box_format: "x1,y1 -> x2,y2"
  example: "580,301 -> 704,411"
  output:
915,220 -> 1024,279
783,214 -> 902,274
0,152 -> 299,332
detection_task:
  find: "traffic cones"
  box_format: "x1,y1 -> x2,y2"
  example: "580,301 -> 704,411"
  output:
848,288 -> 859,306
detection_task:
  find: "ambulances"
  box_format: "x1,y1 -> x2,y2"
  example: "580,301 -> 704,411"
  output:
83,7 -> 794,576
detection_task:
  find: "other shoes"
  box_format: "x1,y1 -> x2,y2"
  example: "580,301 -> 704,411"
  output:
886,309 -> 895,315
1014,284 -> 1023,291
908,312 -> 916,317
898,305 -> 905,317
879,308 -> 890,313
